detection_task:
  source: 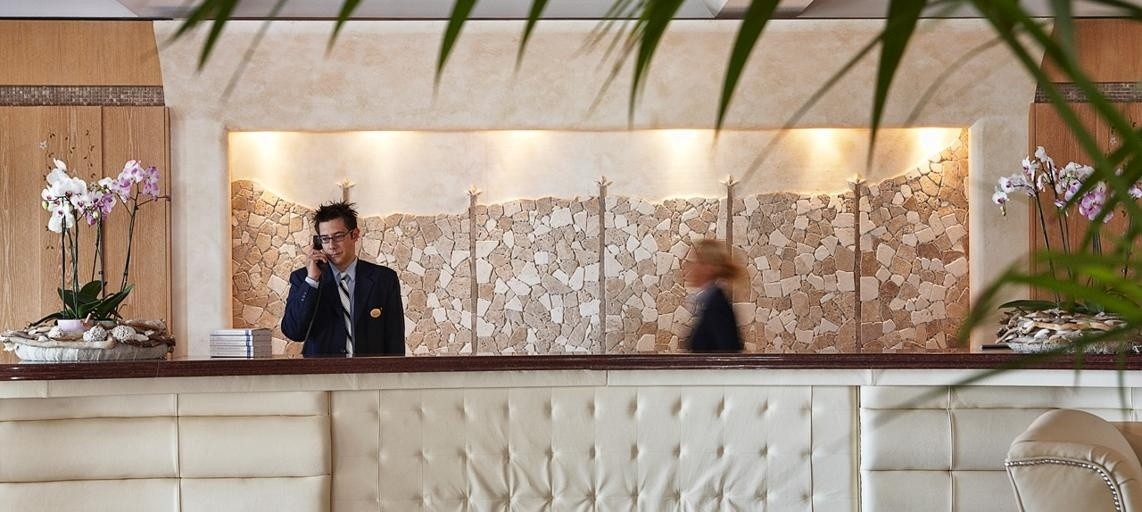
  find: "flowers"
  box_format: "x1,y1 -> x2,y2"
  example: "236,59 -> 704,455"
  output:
26,159 -> 170,326
993,146 -> 1142,317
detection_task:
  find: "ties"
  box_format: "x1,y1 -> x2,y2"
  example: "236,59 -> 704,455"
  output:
337,272 -> 354,358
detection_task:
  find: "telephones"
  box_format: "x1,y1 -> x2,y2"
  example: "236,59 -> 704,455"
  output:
313,235 -> 328,272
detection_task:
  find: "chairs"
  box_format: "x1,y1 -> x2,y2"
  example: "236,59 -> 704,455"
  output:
1006,409 -> 1142,512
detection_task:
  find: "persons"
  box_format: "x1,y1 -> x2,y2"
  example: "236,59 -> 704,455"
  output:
679,238 -> 747,352
281,199 -> 405,357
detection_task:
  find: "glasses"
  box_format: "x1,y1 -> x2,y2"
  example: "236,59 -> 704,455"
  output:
317,228 -> 353,244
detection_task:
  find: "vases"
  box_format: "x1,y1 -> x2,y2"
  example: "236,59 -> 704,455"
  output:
57,320 -> 117,343
